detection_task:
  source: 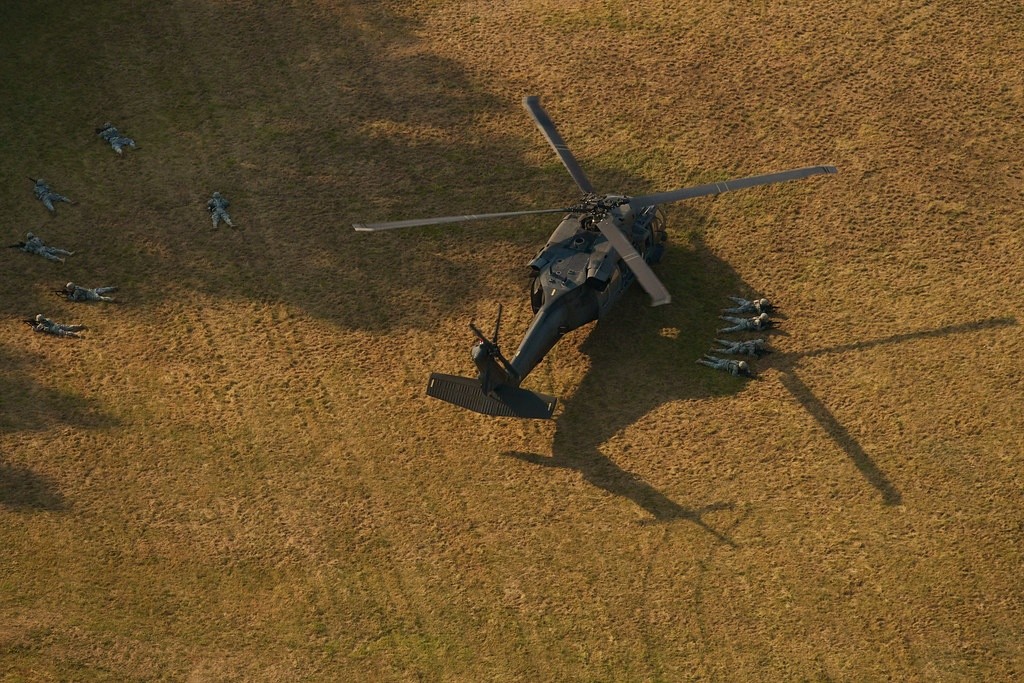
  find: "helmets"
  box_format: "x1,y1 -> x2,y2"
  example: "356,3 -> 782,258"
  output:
756,339 -> 764,345
760,298 -> 768,305
760,313 -> 768,320
739,361 -> 747,370
27,232 -> 34,239
36,314 -> 44,322
37,179 -> 44,186
213,192 -> 221,198
66,282 -> 75,290
104,122 -> 111,129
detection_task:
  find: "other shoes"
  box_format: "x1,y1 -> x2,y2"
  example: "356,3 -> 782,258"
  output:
72,201 -> 79,205
62,258 -> 66,267
70,250 -> 76,257
231,225 -> 238,228
121,150 -> 126,160
695,297 -> 733,363
211,228 -> 218,231
81,324 -> 88,330
53,209 -> 57,217
133,146 -> 141,150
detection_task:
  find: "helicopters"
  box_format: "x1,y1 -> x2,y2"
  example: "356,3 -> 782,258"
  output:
352,95 -> 839,419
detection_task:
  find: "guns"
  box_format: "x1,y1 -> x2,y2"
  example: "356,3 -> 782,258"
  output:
761,320 -> 784,327
19,318 -> 39,327
754,348 -> 773,355
25,175 -> 37,184
92,127 -> 104,135
739,370 -> 762,377
51,290 -> 69,299
7,240 -> 27,249
758,304 -> 781,311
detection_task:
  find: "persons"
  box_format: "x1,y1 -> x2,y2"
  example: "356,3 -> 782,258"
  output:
207,191 -> 238,232
33,178 -> 79,218
694,292 -> 774,378
20,231 -> 76,267
95,123 -> 142,160
64,282 -> 120,303
28,313 -> 89,340
